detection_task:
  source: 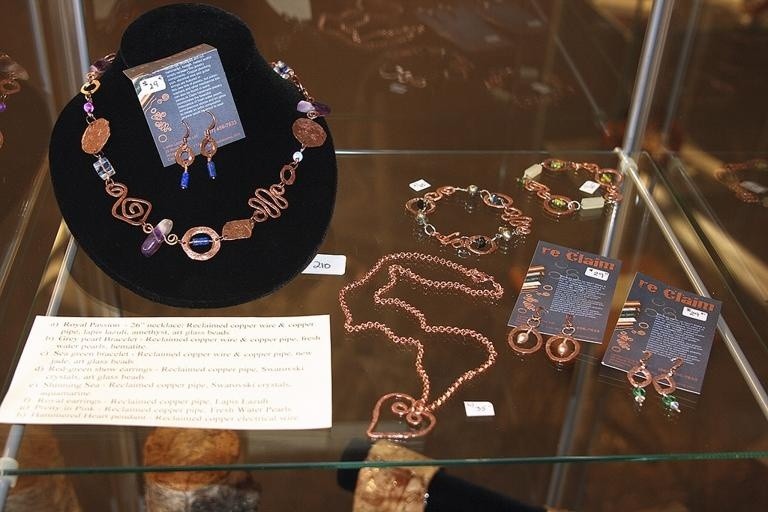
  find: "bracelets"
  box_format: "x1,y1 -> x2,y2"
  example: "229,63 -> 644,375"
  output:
349,438 -> 448,511
712,153 -> 768,214
401,183 -> 534,261
513,156 -> 625,219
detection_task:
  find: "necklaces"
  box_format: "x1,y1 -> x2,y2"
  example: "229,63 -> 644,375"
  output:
311,1 -> 579,110
77,46 -> 335,265
335,251 -> 507,446
0,50 -> 34,154
